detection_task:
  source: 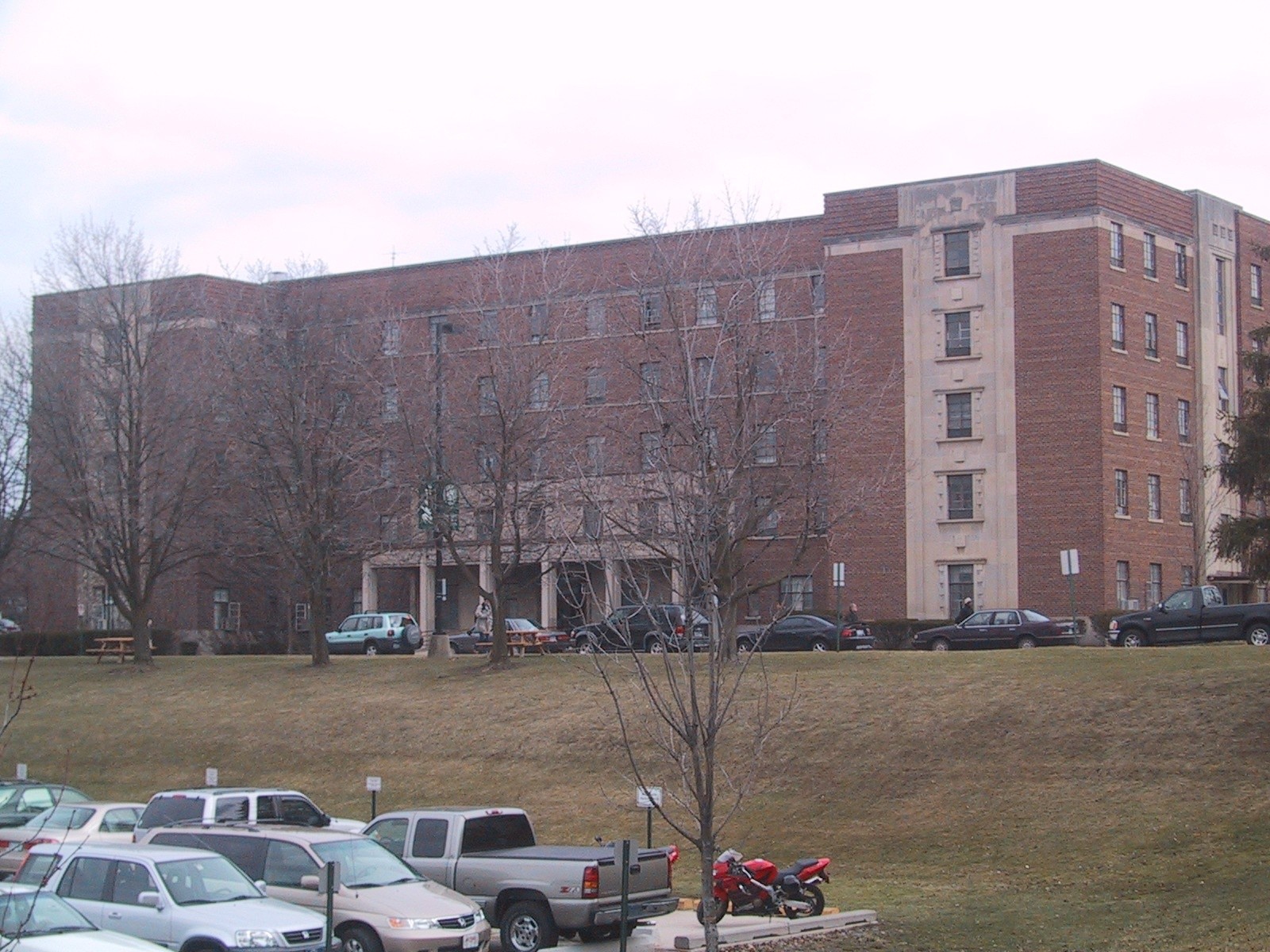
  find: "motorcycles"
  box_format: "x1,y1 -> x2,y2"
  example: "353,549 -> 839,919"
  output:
697,845 -> 831,926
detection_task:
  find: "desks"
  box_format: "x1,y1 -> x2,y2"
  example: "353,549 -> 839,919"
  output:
483,629 -> 545,659
94,637 -> 135,664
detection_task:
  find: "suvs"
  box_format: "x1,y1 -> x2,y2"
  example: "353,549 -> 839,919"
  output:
569,605 -> 711,654
324,612 -> 425,655
134,787 -> 382,866
1,780 -> 122,834
7,844 -> 343,951
131,817 -> 493,950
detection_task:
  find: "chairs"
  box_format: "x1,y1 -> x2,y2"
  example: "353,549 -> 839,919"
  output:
0,904 -> 18,933
114,871 -> 139,889
167,868 -> 195,901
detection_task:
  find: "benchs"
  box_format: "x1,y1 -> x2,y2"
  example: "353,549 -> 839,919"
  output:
475,642 -> 528,648
86,648 -> 135,654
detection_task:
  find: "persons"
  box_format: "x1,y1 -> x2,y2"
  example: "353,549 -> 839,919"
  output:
472,598 -> 493,656
845,604 -> 858,625
954,596 -> 978,625
145,618 -> 154,649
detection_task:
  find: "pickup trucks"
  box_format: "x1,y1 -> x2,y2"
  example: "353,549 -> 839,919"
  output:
1108,585 -> 1270,648
348,806 -> 680,951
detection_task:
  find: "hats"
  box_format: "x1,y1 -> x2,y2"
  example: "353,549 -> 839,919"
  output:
964,597 -> 971,605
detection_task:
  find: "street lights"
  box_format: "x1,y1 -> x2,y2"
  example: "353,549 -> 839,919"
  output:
420,322 -> 461,657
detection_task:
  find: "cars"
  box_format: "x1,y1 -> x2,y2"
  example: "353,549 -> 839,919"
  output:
734,615 -> 877,652
0,883 -> 171,950
0,803 -> 149,882
449,618 -> 569,652
912,609 -> 1079,652
